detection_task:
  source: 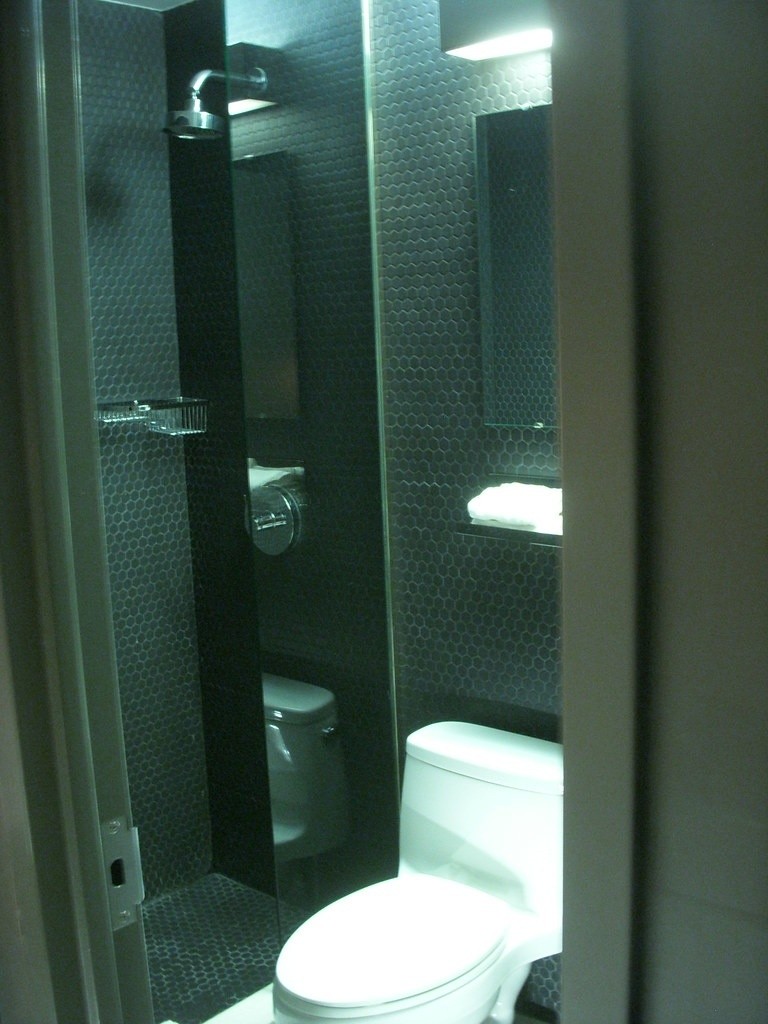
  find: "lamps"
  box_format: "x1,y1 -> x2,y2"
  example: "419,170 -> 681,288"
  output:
436,1 -> 553,62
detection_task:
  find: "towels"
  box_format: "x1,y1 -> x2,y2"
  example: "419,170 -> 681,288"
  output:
466,480 -> 562,533
246,449 -> 305,506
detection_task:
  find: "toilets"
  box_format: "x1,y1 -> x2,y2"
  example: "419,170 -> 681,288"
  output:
268,718 -> 565,1023
262,665 -> 356,872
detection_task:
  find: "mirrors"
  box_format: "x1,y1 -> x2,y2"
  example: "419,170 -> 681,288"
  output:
471,101 -> 559,432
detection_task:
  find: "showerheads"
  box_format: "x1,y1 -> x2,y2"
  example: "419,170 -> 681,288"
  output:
160,102 -> 236,151
